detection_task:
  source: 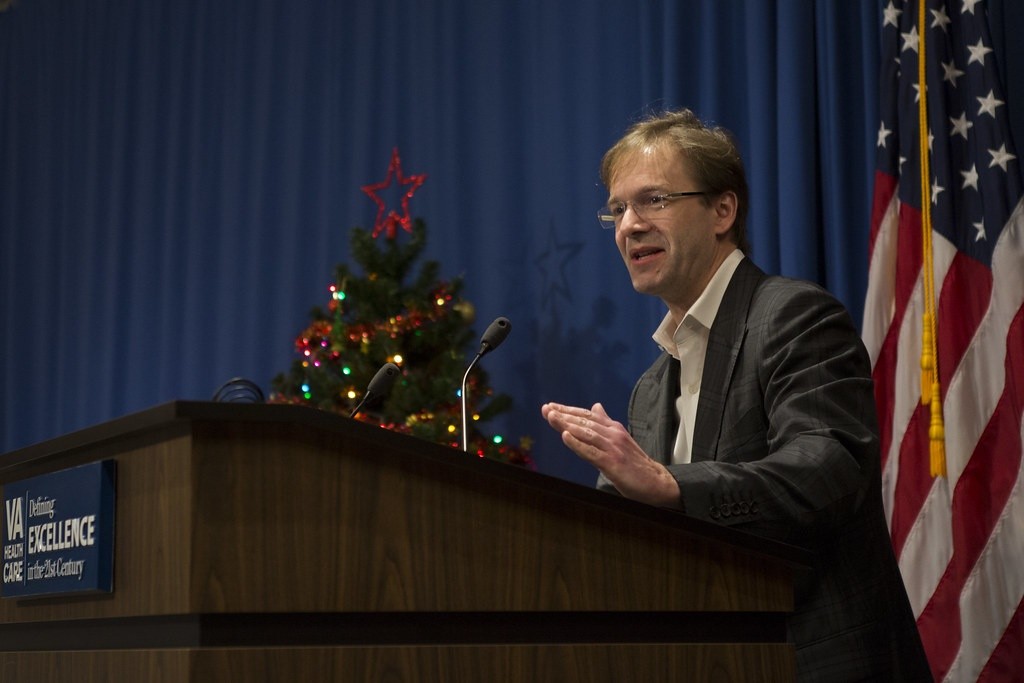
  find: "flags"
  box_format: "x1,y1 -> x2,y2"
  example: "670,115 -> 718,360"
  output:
857,1 -> 1024,683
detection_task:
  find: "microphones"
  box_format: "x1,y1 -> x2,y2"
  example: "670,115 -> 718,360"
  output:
462,317 -> 512,453
352,363 -> 400,418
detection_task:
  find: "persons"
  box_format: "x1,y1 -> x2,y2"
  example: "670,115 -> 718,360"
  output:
538,113 -> 937,683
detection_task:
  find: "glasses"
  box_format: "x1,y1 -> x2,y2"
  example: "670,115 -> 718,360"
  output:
597,191 -> 720,229
